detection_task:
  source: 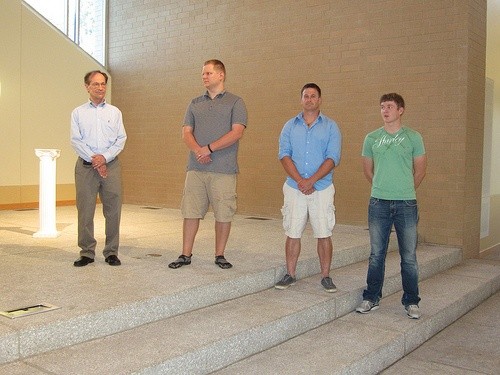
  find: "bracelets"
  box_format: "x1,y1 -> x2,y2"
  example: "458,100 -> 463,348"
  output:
207,144 -> 214,153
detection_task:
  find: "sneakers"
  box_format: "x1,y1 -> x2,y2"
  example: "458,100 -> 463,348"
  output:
405,305 -> 421,319
320,276 -> 337,292
275,274 -> 297,289
215,255 -> 233,269
355,300 -> 380,313
168,254 -> 193,269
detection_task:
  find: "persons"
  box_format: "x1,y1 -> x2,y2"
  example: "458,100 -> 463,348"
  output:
69,70 -> 127,266
168,59 -> 248,269
355,92 -> 428,319
274,83 -> 342,293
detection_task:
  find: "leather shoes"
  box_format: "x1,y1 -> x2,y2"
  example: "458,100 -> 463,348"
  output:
74,256 -> 94,267
105,255 -> 121,266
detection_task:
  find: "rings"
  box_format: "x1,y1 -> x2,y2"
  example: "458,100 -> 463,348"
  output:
199,155 -> 201,157
